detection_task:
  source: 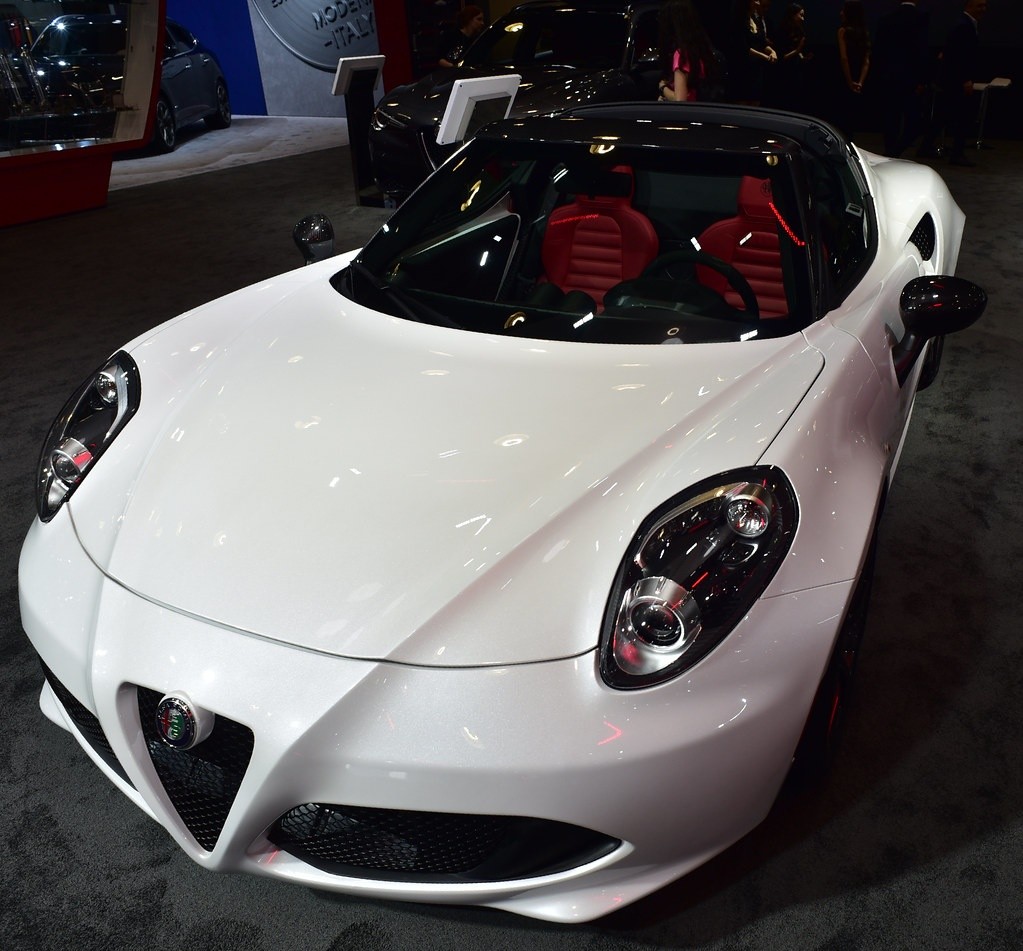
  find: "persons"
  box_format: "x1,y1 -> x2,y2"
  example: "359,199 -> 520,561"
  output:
732,0 -> 872,93
656,0 -> 725,101
914,0 -> 987,166
875,0 -> 930,155
438,5 -> 484,67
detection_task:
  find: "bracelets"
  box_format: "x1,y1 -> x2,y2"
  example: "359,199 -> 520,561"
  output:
660,84 -> 672,98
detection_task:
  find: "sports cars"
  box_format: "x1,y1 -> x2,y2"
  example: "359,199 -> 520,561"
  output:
17,105 -> 966,932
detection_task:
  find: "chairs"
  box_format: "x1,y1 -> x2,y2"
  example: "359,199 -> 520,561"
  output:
538,166 -> 659,315
694,177 -> 810,316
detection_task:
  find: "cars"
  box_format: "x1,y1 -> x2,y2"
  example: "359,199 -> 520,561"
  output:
28,15 -> 231,155
368,0 -> 662,182
